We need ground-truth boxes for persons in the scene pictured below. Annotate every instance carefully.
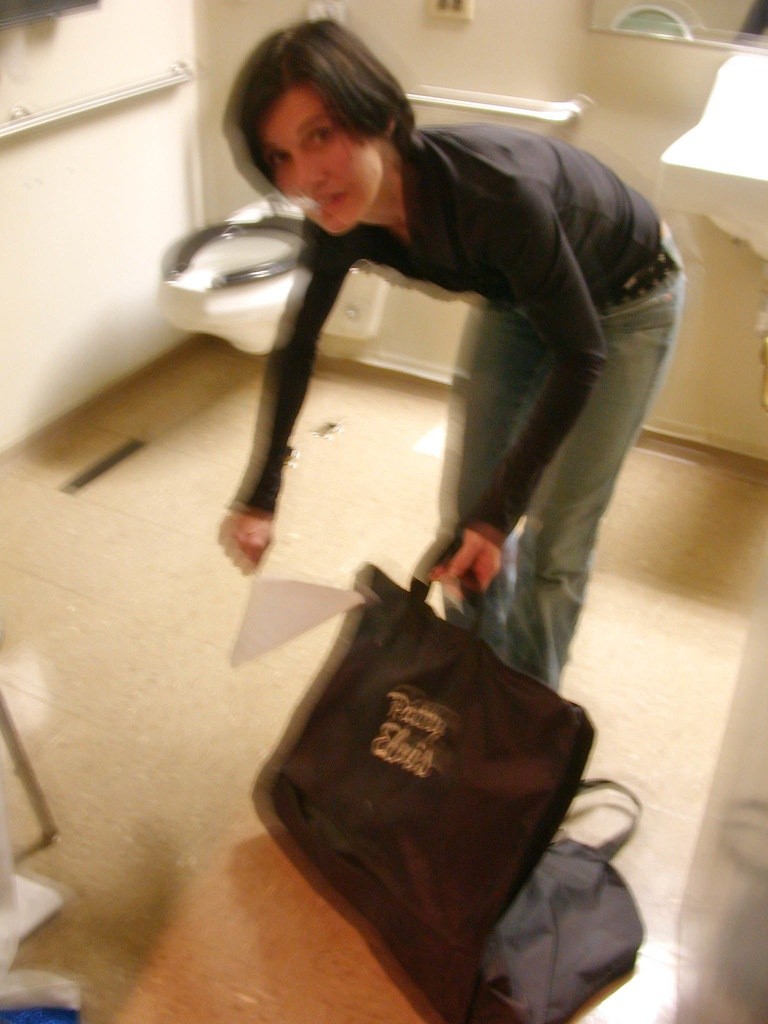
[216,18,685,693]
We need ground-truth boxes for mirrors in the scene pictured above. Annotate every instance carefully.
[590,1,768,55]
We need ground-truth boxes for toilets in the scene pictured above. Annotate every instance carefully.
[154,187,315,357]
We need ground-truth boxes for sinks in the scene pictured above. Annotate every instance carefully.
[653,52,768,265]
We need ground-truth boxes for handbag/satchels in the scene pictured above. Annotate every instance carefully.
[269,541,645,1023]
